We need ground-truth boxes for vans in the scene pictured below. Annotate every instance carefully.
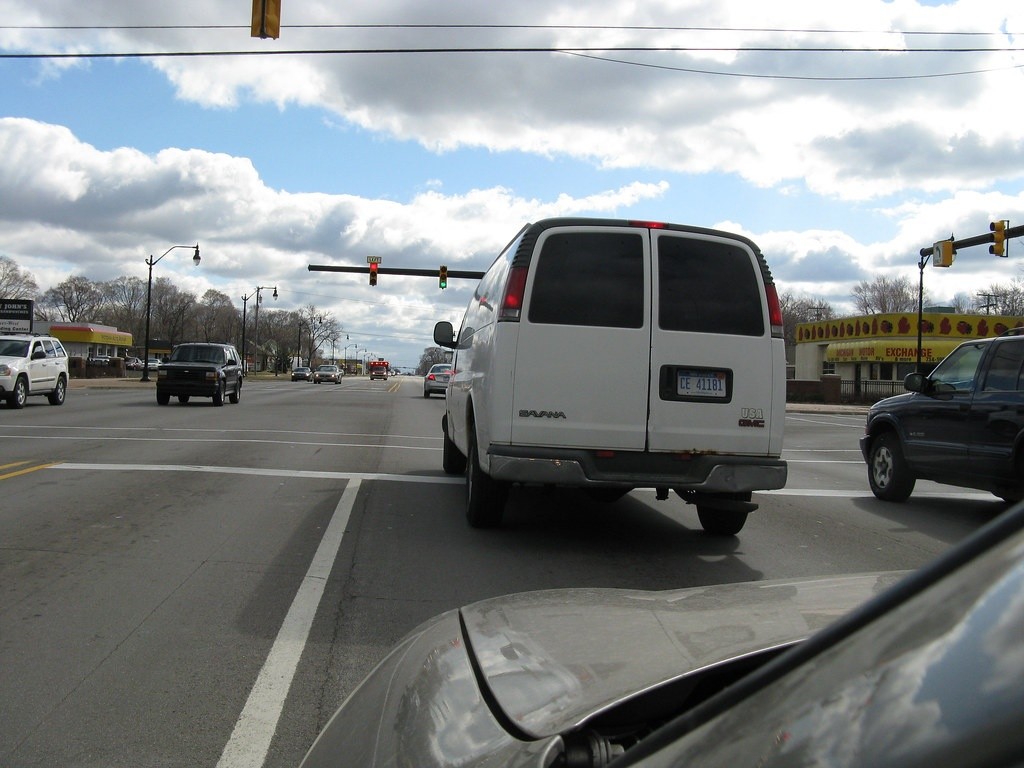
[434,218,788,535]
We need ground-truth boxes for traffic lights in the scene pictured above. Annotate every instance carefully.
[989,221,1005,255]
[370,262,377,285]
[439,266,447,288]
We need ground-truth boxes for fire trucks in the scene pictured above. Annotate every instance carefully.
[369,362,389,380]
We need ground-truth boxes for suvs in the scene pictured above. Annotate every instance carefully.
[0,336,69,408]
[156,343,242,406]
[859,327,1024,502]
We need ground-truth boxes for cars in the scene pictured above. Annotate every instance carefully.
[297,502,1024,768]
[314,365,342,384]
[424,364,452,398]
[93,356,161,371]
[292,367,313,382]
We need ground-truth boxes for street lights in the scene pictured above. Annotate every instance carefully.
[332,334,349,364]
[241,286,278,378]
[140,243,201,381]
[356,349,376,374]
[345,344,357,374]
[297,317,323,367]
